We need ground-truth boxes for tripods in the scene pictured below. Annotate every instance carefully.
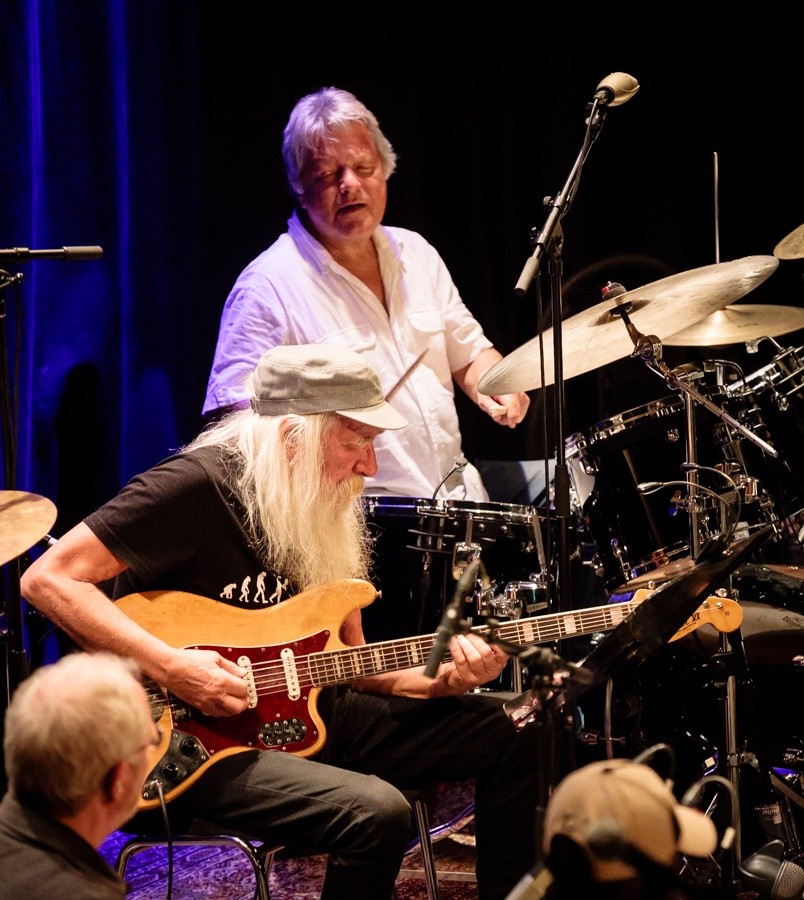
[680,573,804,878]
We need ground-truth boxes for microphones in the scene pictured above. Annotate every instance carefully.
[739,853,804,900]
[423,560,477,680]
[594,72,640,108]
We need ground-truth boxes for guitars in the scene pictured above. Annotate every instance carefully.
[112,578,745,810]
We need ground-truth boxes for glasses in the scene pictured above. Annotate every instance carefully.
[122,727,166,755]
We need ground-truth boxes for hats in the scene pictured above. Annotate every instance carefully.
[543,760,717,881]
[250,343,410,430]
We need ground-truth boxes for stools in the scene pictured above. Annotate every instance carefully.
[115,837,295,900]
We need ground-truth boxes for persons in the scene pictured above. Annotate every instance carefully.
[19,356,557,900]
[0,651,162,900]
[504,758,717,900]
[203,88,529,645]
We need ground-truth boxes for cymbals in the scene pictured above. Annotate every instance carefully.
[773,223,804,260]
[660,305,804,347]
[477,255,780,396]
[0,490,57,567]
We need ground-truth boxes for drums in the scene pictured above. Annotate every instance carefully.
[361,496,558,695]
[555,345,804,808]
